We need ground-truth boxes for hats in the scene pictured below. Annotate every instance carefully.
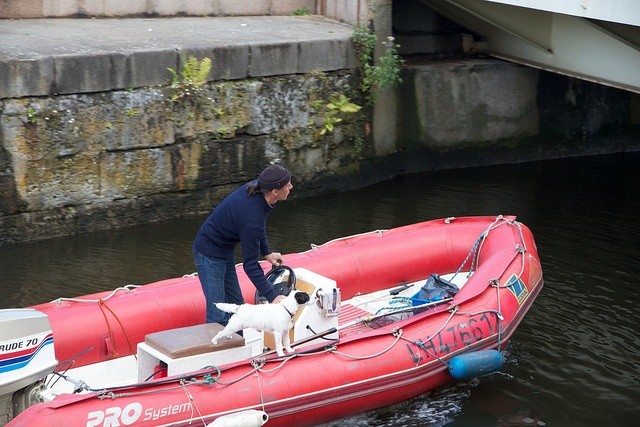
[258,164,290,190]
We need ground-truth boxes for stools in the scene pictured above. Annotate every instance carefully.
[135,322,253,384]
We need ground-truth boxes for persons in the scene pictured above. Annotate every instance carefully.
[192,166,294,324]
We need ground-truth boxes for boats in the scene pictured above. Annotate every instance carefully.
[0,214,544,427]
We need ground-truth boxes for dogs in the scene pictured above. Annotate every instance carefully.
[210,289,318,358]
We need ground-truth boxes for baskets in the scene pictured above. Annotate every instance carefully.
[409,289,447,315]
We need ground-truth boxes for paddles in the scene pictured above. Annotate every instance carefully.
[87,298,456,391]
